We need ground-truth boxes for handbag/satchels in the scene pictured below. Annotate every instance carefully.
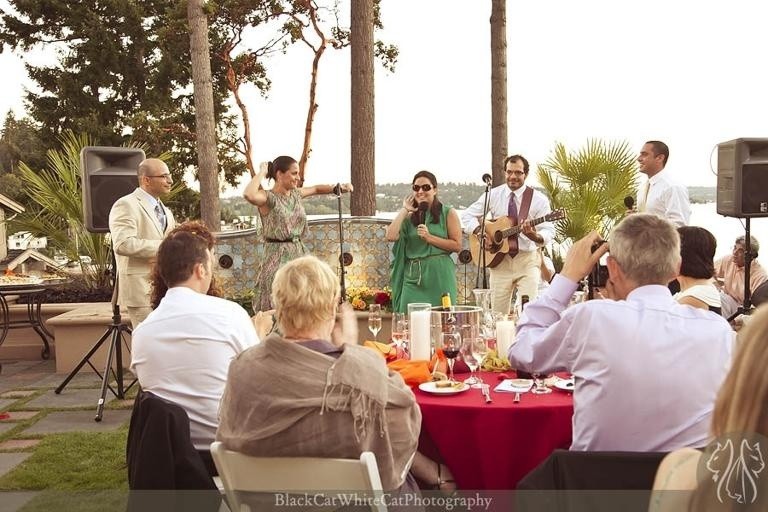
[364,339,396,360]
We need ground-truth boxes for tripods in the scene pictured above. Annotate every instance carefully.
[54,251,138,422]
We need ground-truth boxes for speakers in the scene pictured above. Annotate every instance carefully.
[80,146,147,233]
[717,137,768,217]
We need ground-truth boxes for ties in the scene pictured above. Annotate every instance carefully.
[155,203,164,231]
[640,180,650,212]
[508,191,519,259]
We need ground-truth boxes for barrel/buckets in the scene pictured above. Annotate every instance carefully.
[430,305,484,359]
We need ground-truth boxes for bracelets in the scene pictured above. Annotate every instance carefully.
[402,207,410,213]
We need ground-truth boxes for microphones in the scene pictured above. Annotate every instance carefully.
[419,201,428,240]
[483,174,493,187]
[333,183,343,195]
[624,196,634,210]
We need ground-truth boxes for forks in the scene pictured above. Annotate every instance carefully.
[479,383,494,403]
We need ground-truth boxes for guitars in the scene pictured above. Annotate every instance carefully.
[469,207,566,267]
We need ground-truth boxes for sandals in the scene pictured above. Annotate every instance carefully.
[427,463,462,505]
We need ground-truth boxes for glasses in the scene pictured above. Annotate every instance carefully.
[150,173,173,180]
[412,184,433,192]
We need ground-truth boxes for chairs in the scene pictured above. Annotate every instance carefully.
[126,385,230,511]
[211,441,388,511]
[514,450,711,512]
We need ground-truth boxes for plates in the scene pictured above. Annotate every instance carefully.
[553,379,576,391]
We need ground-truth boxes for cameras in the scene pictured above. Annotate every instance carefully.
[591,241,610,287]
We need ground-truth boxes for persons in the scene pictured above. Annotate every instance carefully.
[147,219,225,308]
[108,158,174,329]
[385,171,462,314]
[129,230,258,474]
[243,155,353,317]
[218,255,457,512]
[462,140,768,512]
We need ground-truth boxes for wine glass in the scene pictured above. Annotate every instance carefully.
[367,287,554,395]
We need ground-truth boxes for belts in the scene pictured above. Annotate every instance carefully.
[267,237,300,244]
